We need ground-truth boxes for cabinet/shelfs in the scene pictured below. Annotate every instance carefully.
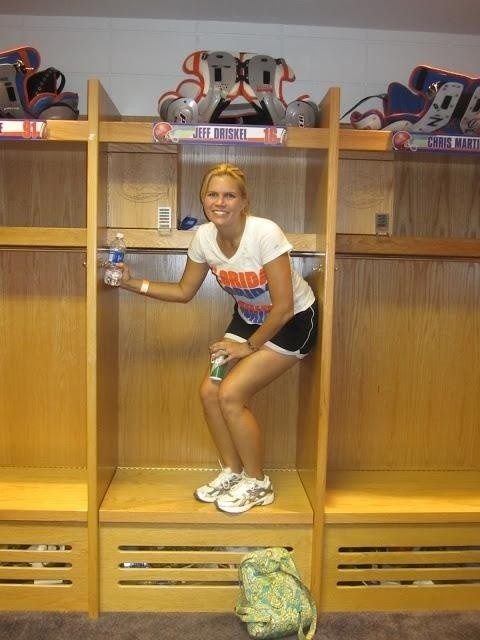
[0,78,480,619]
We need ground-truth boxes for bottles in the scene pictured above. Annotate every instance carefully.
[104,234,126,288]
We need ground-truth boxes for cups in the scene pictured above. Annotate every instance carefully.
[209,347,227,381]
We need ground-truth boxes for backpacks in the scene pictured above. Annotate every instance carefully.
[233,546,318,639]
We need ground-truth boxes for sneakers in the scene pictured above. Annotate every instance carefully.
[193,462,245,504]
[214,473,274,514]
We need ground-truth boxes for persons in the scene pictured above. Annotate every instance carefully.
[101,161,319,517]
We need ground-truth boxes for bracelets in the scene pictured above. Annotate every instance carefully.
[247,337,259,354]
[140,275,150,298]
